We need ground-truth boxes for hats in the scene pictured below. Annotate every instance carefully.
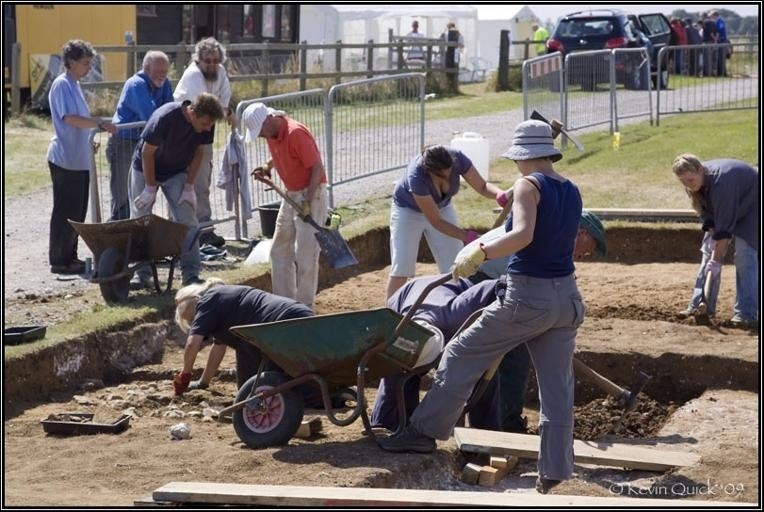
[500,118,563,164]
[241,102,271,144]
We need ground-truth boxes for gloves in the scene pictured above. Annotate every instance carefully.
[173,371,193,396]
[250,163,271,183]
[134,184,157,213]
[187,380,209,392]
[297,199,311,223]
[463,230,481,246]
[450,243,490,283]
[177,183,197,211]
[707,234,729,257]
[495,187,514,208]
[704,259,722,280]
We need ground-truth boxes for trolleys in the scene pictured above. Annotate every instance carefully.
[66,213,217,307]
[218,269,454,452]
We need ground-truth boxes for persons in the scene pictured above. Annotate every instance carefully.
[449,210,607,433]
[385,143,507,298]
[669,11,729,76]
[531,24,549,57]
[104,49,175,221]
[240,101,327,309]
[126,91,225,288]
[672,152,758,327]
[404,21,424,68]
[376,118,585,495]
[173,37,231,249]
[174,278,316,395]
[442,22,464,68]
[361,273,506,435]
[45,39,119,274]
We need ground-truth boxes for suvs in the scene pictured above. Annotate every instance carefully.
[545,7,675,92]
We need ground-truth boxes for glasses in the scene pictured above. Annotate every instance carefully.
[202,58,221,64]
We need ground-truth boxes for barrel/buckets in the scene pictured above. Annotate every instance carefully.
[450,130,490,183]
[257,203,281,238]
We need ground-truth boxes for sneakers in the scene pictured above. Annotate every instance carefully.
[376,422,435,454]
[730,313,757,324]
[535,477,560,494]
[127,270,151,289]
[181,275,205,286]
[679,304,696,318]
[199,231,226,248]
[50,257,87,275]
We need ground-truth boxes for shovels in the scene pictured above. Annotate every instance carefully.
[251,166,360,272]
